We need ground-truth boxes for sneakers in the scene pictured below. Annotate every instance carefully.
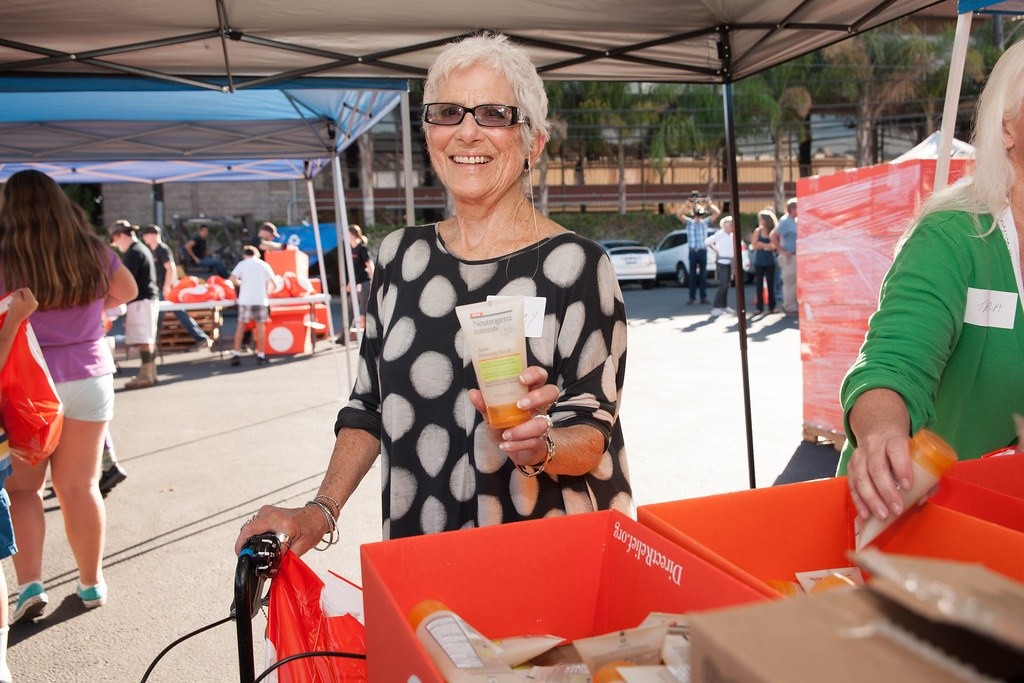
[9,583,49,625]
[100,465,127,499]
[76,581,107,608]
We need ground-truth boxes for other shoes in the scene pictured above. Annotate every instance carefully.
[755,310,774,314]
[257,357,267,366]
[687,299,694,304]
[335,340,345,346]
[126,371,158,388]
[231,356,239,365]
[712,308,736,317]
[786,313,798,317]
[701,300,710,304]
[189,338,213,352]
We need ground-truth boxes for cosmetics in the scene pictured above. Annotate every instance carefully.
[455,296,533,428]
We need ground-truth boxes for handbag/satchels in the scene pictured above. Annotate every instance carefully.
[264,549,367,683]
[0,293,62,464]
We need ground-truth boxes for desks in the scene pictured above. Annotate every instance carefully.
[160,293,329,355]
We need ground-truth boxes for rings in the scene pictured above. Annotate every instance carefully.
[274,533,293,547]
[536,406,547,412]
[534,415,553,434]
[239,516,255,530]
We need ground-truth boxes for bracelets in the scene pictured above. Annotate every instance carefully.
[303,503,333,551]
[314,495,341,522]
[308,501,340,544]
[314,502,334,517]
[515,436,556,477]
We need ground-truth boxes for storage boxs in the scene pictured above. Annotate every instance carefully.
[361,452,1024,683]
[249,278,328,355]
[266,250,310,279]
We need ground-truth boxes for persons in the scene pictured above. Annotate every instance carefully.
[236,31,638,557]
[676,197,798,317]
[838,44,1023,521]
[0,170,376,683]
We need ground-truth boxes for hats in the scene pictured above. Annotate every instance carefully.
[141,224,161,233]
[107,219,140,235]
[260,221,280,239]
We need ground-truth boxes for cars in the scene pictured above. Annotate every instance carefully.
[596,238,657,289]
[648,228,751,286]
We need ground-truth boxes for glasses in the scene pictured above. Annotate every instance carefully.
[110,237,115,243]
[423,102,529,127]
